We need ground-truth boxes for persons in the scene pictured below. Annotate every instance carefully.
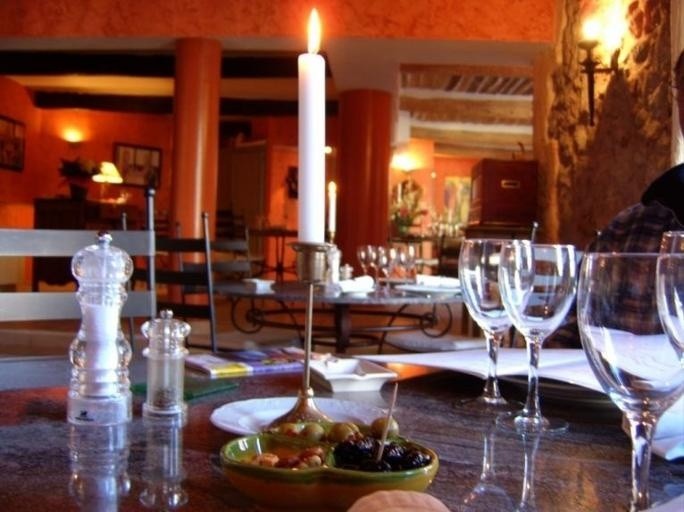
[575,49,684,335]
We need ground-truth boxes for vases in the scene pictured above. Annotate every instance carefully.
[399,224,407,239]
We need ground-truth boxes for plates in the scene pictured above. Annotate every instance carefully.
[298,359,399,395]
[209,392,399,442]
[392,284,459,300]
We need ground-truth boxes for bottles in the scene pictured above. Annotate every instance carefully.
[63,234,134,428]
[66,424,133,512]
[340,263,354,280]
[139,309,193,419]
[137,414,191,511]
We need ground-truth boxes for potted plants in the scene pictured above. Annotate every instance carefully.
[58,155,97,197]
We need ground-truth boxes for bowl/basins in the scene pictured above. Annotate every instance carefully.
[225,420,440,502]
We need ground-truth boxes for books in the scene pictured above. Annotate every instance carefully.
[183,343,319,380]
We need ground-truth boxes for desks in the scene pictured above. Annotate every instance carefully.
[2,370,684,509]
[191,275,461,353]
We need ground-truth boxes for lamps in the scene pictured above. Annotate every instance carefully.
[578,24,620,131]
[94,162,123,191]
[57,131,86,148]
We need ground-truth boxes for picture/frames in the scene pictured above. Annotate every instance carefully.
[1,113,25,172]
[110,143,160,190]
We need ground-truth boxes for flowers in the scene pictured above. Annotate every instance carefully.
[391,204,426,223]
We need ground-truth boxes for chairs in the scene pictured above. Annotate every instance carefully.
[1,193,297,418]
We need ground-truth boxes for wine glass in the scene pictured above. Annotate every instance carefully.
[653,225,683,349]
[354,242,416,295]
[515,429,554,509]
[494,243,579,437]
[454,405,515,512]
[574,249,683,511]
[454,236,531,417]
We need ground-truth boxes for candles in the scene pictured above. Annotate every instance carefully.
[296,9,328,244]
[328,181,338,232]
